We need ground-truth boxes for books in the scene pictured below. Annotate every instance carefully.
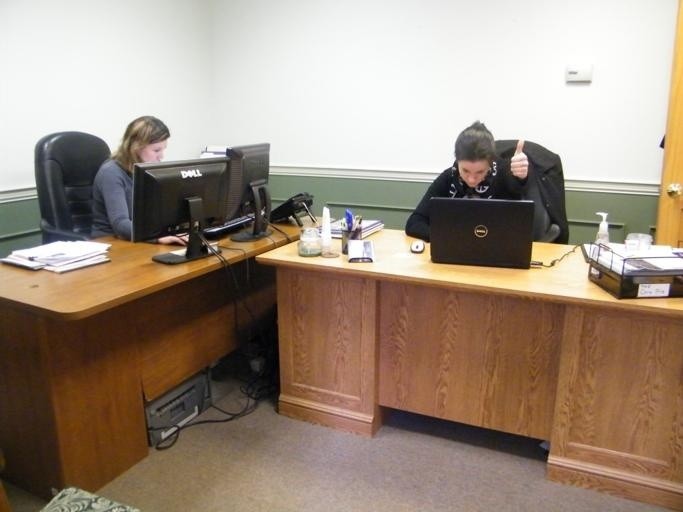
[0,238,114,275]
[315,218,385,240]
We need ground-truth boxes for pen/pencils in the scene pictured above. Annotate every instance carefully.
[342,208,362,252]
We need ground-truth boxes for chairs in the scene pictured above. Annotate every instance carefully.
[33,131,114,247]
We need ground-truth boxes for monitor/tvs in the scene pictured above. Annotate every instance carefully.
[132,155,232,265]
[224,142,273,242]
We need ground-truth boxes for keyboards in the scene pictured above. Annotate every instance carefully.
[203,210,265,239]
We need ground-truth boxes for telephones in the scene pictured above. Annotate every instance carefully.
[270,192,314,221]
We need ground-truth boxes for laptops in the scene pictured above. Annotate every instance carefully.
[428,197,535,269]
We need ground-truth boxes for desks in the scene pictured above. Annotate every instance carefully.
[2,213,336,508]
[254,225,682,511]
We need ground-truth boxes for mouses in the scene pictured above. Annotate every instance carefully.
[411,239,425,252]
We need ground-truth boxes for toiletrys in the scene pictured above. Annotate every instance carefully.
[594,211,610,244]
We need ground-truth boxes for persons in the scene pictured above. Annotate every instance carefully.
[404,120,531,245]
[89,115,191,247]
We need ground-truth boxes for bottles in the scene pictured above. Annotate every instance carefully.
[298,228,321,257]
